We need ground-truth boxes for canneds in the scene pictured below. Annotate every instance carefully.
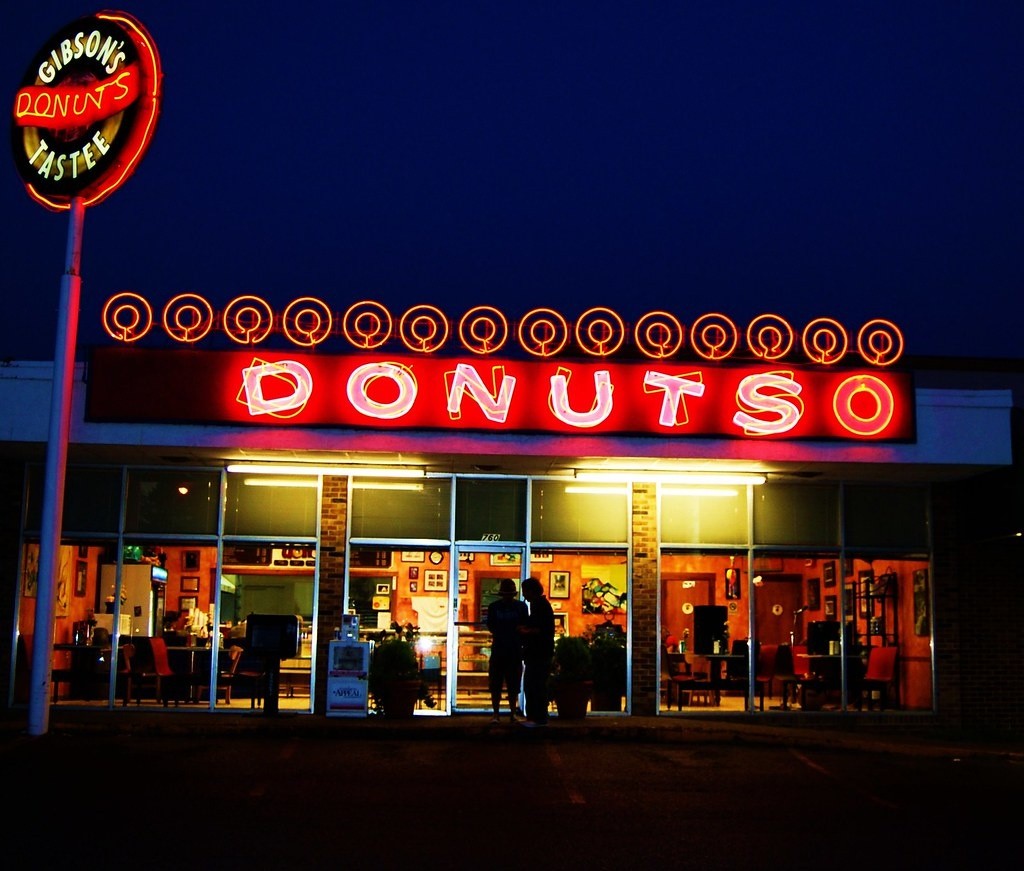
[679,641,685,654]
[829,641,835,656]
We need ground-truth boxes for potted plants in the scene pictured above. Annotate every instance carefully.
[552,630,629,717]
[368,641,439,718]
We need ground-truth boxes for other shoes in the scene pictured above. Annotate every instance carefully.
[490,717,501,727]
[510,717,520,726]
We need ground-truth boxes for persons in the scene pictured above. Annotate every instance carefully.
[516,578,555,728]
[486,578,528,724]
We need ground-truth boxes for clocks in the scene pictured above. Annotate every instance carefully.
[429,552,444,564]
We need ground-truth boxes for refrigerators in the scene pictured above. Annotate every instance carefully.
[98,565,168,640]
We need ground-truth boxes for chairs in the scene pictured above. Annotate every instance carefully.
[663,642,903,713]
[52,637,244,704]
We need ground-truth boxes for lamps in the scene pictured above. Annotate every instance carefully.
[225,464,427,477]
[574,470,768,484]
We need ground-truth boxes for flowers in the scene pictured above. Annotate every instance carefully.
[106,587,127,602]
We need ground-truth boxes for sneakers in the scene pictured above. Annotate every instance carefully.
[520,721,548,727]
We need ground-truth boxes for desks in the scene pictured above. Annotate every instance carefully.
[697,654,743,707]
[166,646,236,703]
[54,644,107,701]
[799,654,868,711]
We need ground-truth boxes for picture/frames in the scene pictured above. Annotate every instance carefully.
[552,612,570,639]
[490,553,522,566]
[182,549,201,572]
[824,595,837,621]
[824,560,836,587]
[549,571,570,599]
[530,553,554,563]
[458,569,468,582]
[858,569,875,617]
[459,553,475,562]
[424,569,448,592]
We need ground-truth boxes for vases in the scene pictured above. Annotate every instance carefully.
[105,602,115,613]
